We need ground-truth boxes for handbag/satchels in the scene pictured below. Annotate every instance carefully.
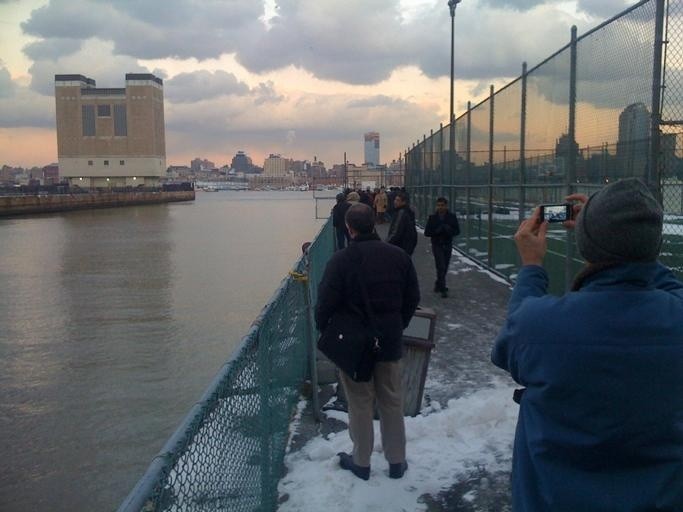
[318,312,379,382]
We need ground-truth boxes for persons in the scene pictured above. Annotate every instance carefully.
[421,195,460,300]
[308,201,427,483]
[483,175,681,509]
[329,179,419,281]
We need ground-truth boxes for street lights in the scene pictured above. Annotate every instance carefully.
[446,0,464,214]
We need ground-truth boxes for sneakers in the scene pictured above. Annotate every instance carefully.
[337,452,369,480]
[434,287,448,298]
[391,461,408,477]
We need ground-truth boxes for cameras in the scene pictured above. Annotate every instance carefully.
[539,202,575,224]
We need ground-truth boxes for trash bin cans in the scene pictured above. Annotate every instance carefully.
[369,305,437,420]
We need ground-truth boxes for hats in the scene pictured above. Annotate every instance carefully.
[575,177,665,261]
[347,192,360,201]
